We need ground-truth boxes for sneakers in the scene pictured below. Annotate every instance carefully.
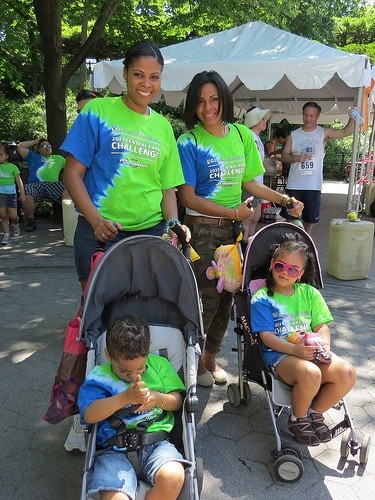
[309,412,332,442]
[11,223,21,237]
[64,413,87,453]
[287,413,320,446]
[2,233,12,245]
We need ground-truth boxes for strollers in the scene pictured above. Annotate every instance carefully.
[76,220,208,499]
[231,198,372,485]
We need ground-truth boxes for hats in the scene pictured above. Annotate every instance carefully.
[244,107,272,128]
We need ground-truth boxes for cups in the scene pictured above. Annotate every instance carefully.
[306,146,312,160]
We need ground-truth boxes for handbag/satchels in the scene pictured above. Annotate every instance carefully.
[263,154,277,175]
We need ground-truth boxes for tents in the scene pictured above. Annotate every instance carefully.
[93,20,375,214]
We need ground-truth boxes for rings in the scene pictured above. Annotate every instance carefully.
[101,233,105,237]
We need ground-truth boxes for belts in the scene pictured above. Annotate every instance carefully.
[183,214,235,227]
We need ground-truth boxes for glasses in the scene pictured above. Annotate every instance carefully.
[76,90,98,97]
[272,257,304,279]
[38,144,52,149]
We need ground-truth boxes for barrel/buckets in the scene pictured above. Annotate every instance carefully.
[327,217,374,280]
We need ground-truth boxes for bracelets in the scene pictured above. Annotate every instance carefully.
[281,193,289,206]
[166,217,180,231]
[234,209,239,220]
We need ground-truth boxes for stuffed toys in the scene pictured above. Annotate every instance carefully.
[287,329,332,365]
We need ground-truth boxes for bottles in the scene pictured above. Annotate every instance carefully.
[347,106,365,125]
[261,208,281,223]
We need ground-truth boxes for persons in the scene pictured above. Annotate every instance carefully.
[75,89,103,115]
[0,138,67,246]
[59,42,191,455]
[178,70,305,387]
[282,102,356,234]
[76,315,187,500]
[248,232,357,447]
[241,102,287,241]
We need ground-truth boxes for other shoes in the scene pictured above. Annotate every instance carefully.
[201,356,228,383]
[243,230,250,243]
[25,219,36,231]
[197,371,215,386]
